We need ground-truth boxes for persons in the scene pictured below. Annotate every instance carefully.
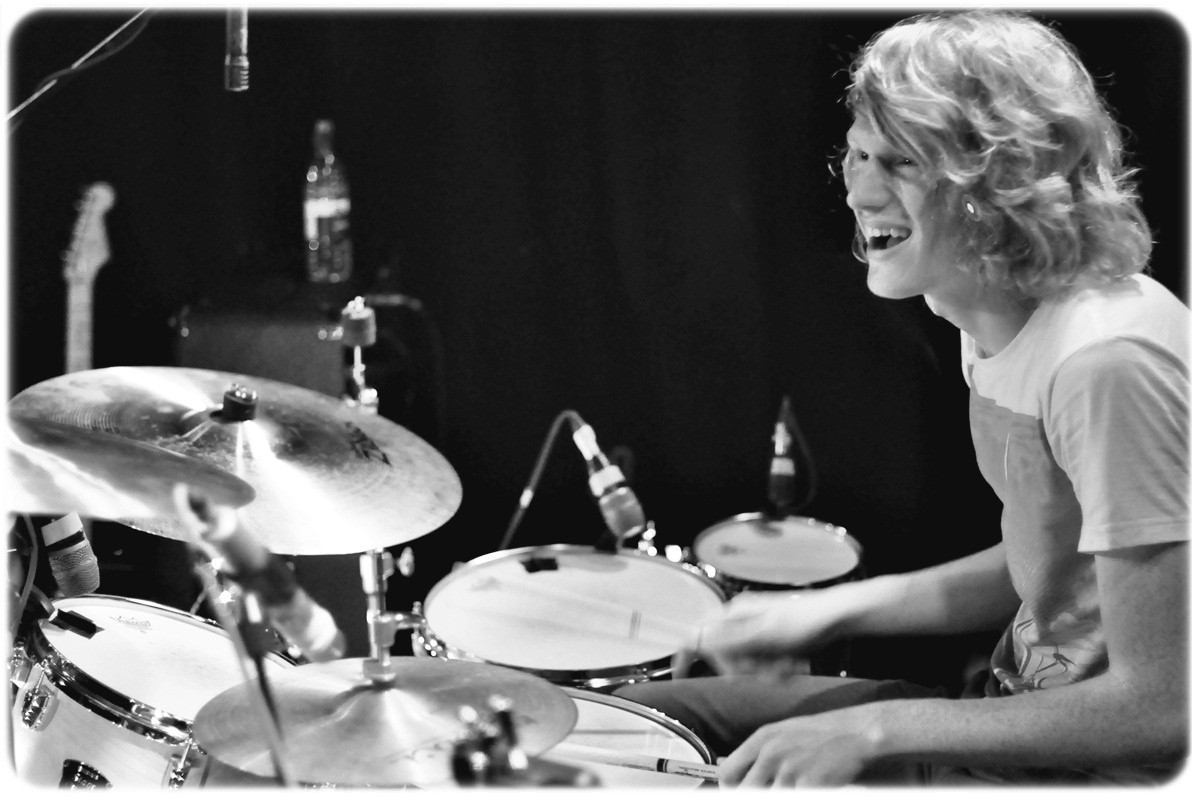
[612,13,1190,786]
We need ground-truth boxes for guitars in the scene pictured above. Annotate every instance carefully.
[61,181,118,372]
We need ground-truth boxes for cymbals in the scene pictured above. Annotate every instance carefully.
[194,647,579,787]
[17,365,466,556]
[3,408,259,523]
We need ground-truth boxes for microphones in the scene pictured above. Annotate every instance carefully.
[186,487,347,660]
[566,412,643,537]
[39,515,100,598]
[226,8,250,92]
[768,404,795,505]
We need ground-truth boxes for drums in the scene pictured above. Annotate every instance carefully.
[485,682,713,791]
[693,510,863,591]
[9,591,301,792]
[414,538,729,687]
